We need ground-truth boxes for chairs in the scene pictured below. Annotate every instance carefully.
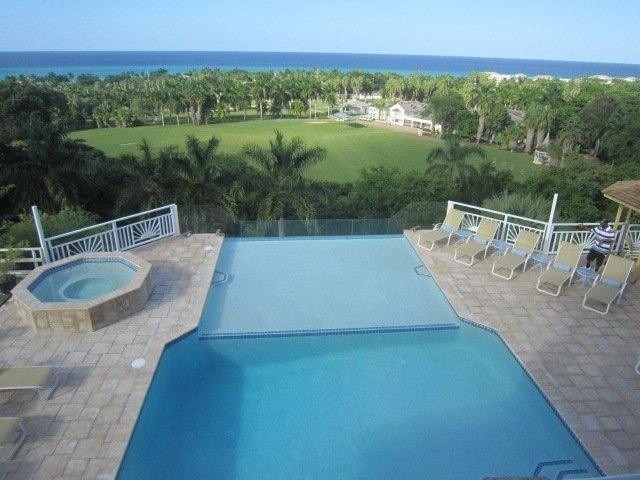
[416,208,635,316]
[0,363,59,463]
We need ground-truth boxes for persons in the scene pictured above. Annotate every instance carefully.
[626,258,640,285]
[577,218,615,271]
